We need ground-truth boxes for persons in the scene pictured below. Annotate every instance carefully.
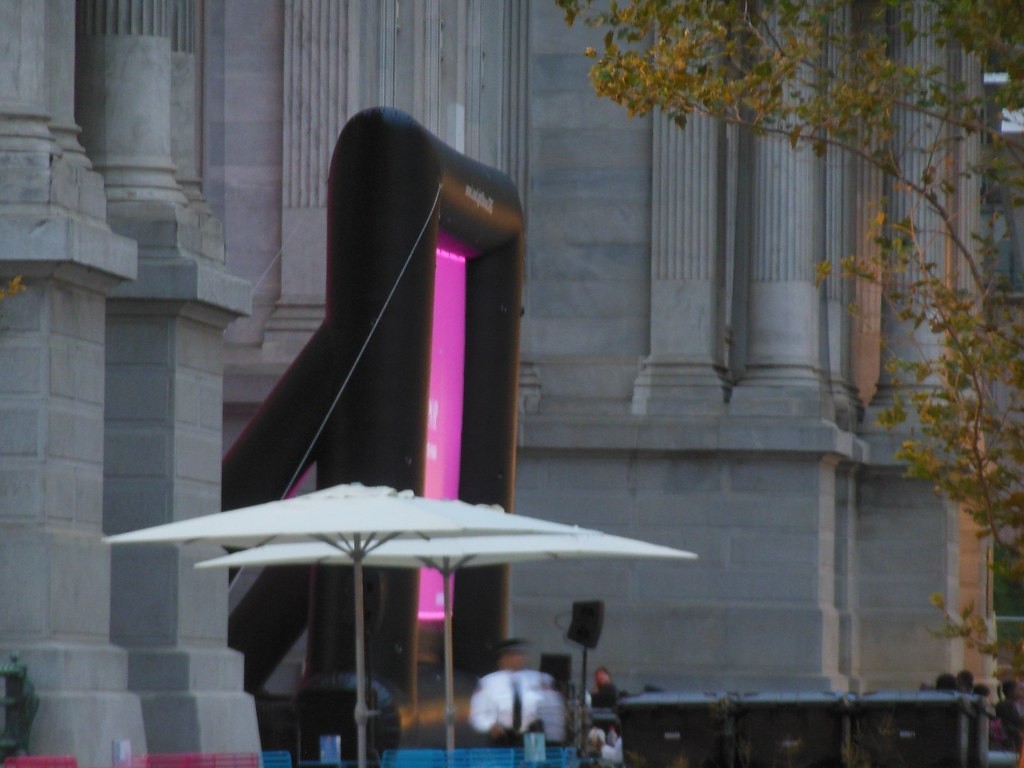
[584,669,623,767]
[468,638,558,748]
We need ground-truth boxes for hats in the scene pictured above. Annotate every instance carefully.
[495,638,530,654]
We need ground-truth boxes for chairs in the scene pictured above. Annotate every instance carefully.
[4,746,595,768]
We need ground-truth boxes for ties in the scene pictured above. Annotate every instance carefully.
[513,680,521,731]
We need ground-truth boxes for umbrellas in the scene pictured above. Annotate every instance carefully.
[192,530,700,767]
[103,481,587,767]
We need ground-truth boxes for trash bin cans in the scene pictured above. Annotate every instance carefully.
[621,691,990,768]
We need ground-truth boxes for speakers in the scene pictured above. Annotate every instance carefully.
[567,599,604,650]
[539,652,570,683]
